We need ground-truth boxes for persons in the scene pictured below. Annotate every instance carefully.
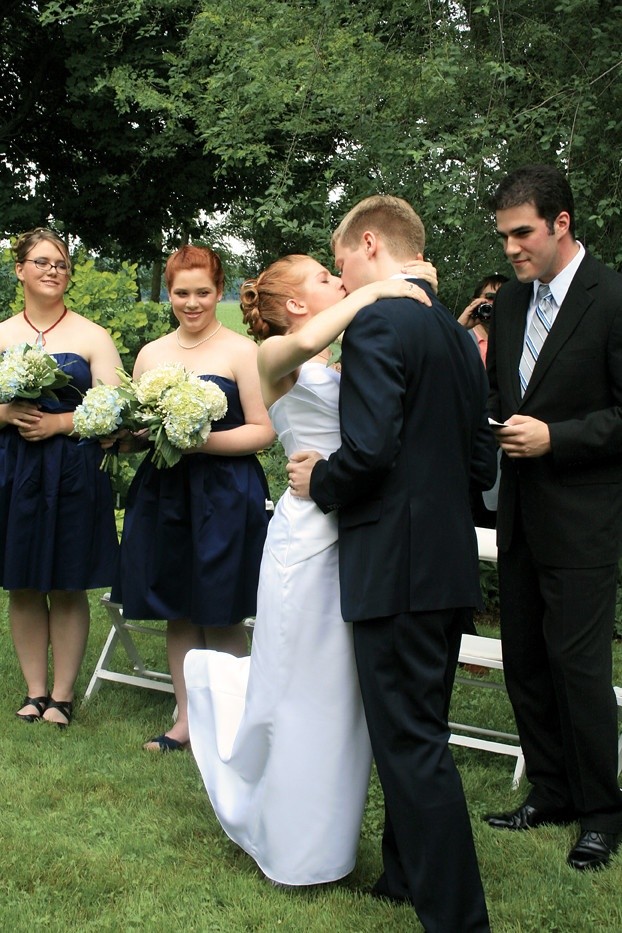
[284,194,498,933]
[456,274,504,529]
[457,619,490,678]
[482,165,622,867]
[0,228,126,727]
[183,254,439,886]
[100,245,275,754]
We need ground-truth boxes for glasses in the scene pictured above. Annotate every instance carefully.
[23,257,71,275]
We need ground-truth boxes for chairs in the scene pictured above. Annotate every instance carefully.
[79,500,526,790]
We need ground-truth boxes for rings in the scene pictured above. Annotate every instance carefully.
[408,285,413,290]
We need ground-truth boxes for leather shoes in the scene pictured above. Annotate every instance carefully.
[567,828,618,869]
[482,802,573,830]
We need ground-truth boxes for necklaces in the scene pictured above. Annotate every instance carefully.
[23,306,68,348]
[176,324,223,350]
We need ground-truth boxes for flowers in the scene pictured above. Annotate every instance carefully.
[0,331,227,475]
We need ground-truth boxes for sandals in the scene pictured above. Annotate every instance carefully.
[143,732,189,753]
[15,688,74,729]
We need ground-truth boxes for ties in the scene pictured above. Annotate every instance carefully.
[519,284,553,401]
